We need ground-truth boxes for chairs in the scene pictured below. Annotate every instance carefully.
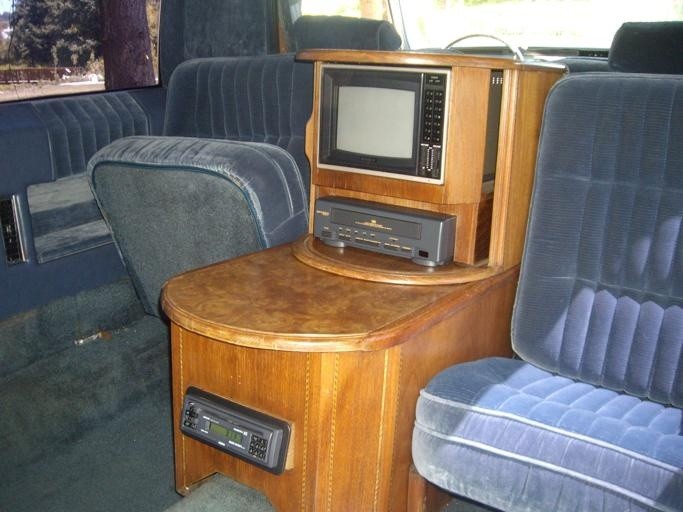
[87,134,307,316]
[159,51,316,190]
[287,14,401,58]
[407,21,683,511]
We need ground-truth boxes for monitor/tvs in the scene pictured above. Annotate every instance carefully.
[317,64,450,186]
